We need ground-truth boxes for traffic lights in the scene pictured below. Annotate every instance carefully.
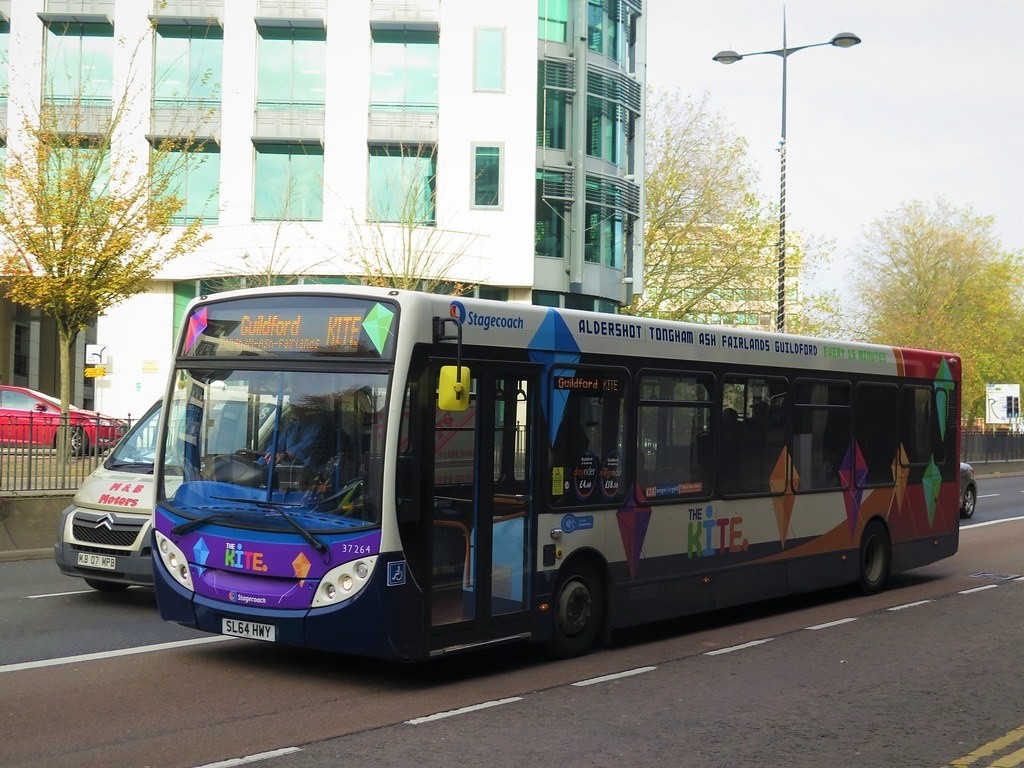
[1006,396,1012,417]
[1013,397,1019,417]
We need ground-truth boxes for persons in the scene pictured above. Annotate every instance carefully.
[722,400,790,449]
[255,391,327,469]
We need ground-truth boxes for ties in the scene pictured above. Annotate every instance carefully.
[292,425,304,444]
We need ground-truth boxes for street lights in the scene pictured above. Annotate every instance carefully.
[712,3,862,335]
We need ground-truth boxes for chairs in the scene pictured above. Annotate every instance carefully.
[691,429,741,489]
[310,412,353,471]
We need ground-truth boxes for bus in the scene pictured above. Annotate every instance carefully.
[150,283,962,665]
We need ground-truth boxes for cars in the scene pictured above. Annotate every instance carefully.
[0,385,127,457]
[960,462,978,518]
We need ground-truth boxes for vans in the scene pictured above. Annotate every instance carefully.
[55,385,475,593]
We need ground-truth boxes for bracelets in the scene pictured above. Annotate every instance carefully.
[282,451,288,459]
